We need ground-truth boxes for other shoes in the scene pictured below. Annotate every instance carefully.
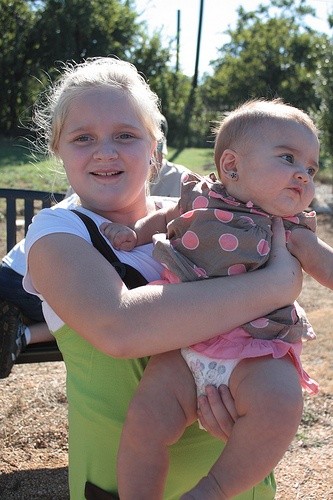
[16,324,26,355]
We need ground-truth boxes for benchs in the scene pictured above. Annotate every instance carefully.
[0,188,66,365]
